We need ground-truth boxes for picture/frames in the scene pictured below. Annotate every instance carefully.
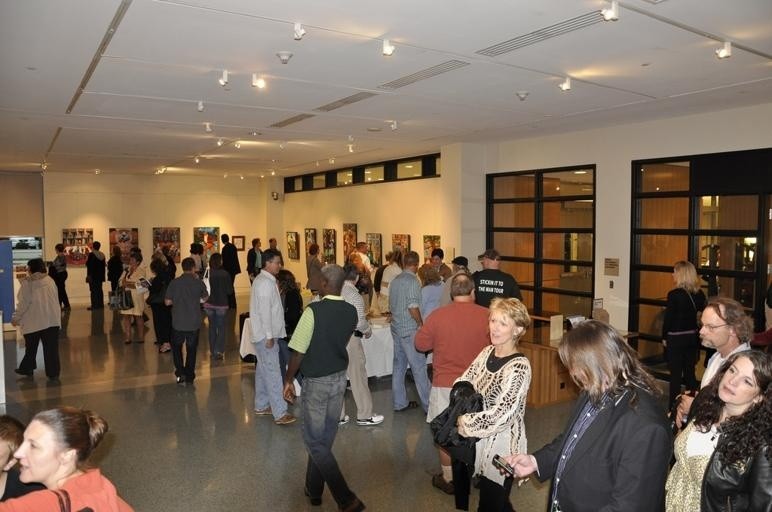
[232,234,246,253]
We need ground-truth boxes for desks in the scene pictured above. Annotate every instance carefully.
[360,317,435,379]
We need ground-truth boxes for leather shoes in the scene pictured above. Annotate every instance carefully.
[47,375,58,381]
[14,368,34,376]
[120,314,225,362]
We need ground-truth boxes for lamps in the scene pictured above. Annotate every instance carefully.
[276,52,292,65]
[219,71,229,87]
[294,23,306,40]
[517,92,529,102]
[252,74,266,89]
[382,40,395,56]
[223,170,276,181]
[391,121,400,134]
[198,102,206,113]
[601,2,620,22]
[316,145,353,167]
[558,78,572,92]
[206,123,241,149]
[157,166,168,177]
[194,157,200,165]
[716,42,732,59]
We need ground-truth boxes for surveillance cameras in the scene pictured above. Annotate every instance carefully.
[271,192,278,200]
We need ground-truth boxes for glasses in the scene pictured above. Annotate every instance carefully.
[700,322,733,331]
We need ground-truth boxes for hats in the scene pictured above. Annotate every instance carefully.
[478,249,501,262]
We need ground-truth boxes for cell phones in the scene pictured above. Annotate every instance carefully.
[494,454,514,475]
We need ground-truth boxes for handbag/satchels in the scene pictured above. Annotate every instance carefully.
[200,267,211,303]
[108,279,135,311]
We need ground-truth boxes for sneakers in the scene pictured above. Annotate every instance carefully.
[431,472,455,495]
[338,414,350,425]
[176,373,194,386]
[394,401,420,412]
[338,497,366,512]
[303,485,322,506]
[253,407,273,415]
[356,412,385,426]
[273,414,298,425]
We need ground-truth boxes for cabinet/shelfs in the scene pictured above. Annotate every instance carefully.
[510,325,644,411]
